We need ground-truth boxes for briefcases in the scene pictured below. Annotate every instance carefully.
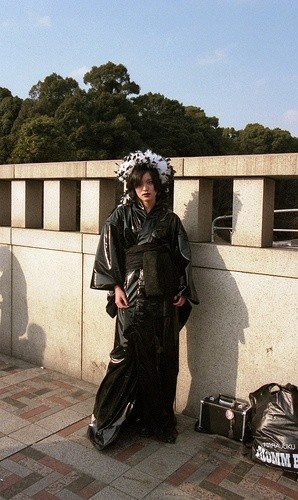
[200,392,251,442]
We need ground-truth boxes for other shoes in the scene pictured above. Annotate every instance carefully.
[139,422,152,437]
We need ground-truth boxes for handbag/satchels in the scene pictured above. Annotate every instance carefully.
[249,384,298,474]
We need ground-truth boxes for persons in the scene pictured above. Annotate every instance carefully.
[87,152,199,452]
